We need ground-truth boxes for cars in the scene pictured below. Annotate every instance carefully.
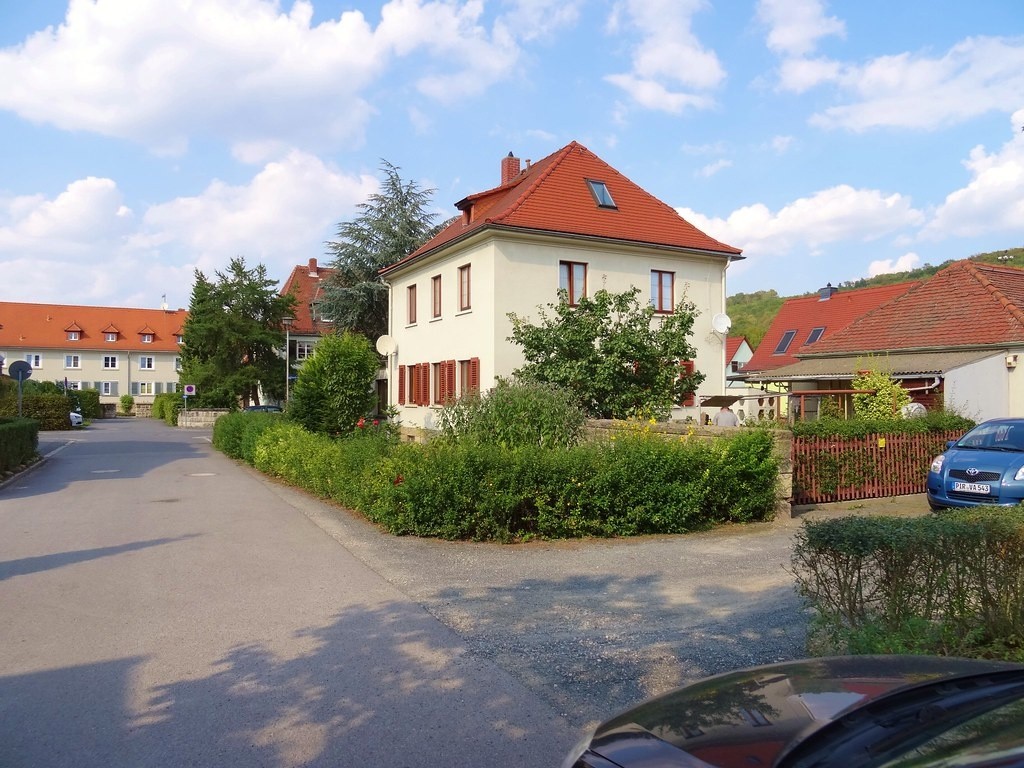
[241,406,282,412]
[927,418,1024,510]
[573,656,1024,768]
[70,413,84,427]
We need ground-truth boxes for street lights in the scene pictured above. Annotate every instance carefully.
[280,316,297,405]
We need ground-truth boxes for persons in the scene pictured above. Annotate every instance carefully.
[712,407,738,427]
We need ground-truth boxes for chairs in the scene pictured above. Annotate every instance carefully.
[1004,428,1024,447]
